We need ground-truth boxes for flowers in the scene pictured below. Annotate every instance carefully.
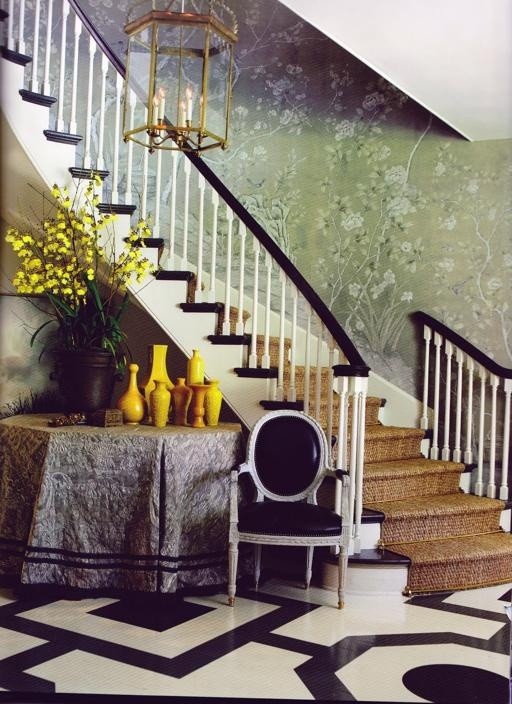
[1,171,166,376]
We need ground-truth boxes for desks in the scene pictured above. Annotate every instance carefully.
[0,409,244,596]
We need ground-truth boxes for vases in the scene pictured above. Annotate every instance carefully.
[75,343,224,428]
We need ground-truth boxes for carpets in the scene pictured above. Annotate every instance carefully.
[129,204,512,598]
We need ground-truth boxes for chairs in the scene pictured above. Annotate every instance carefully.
[225,407,351,610]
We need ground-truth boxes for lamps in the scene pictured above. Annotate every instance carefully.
[120,0,241,160]
